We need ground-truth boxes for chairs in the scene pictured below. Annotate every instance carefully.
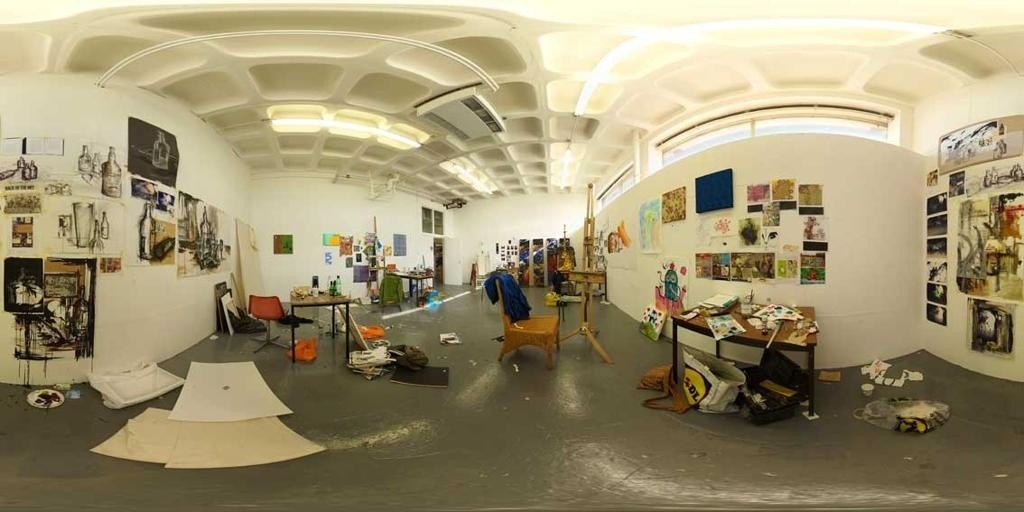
[496,278,560,371]
[248,295,290,354]
[553,270,589,323]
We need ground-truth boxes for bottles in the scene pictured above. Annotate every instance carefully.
[981,121,1023,188]
[329,274,341,297]
[407,265,424,277]
[760,316,769,335]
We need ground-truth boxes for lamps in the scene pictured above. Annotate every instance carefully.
[416,86,506,143]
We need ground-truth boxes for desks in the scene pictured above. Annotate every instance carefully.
[670,302,817,416]
[290,288,354,363]
[567,271,607,302]
[386,272,434,306]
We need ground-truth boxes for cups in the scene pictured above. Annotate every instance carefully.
[312,287,319,298]
[862,384,875,397]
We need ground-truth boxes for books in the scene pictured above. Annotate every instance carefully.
[700,295,738,313]
[390,365,450,387]
[346,349,392,380]
